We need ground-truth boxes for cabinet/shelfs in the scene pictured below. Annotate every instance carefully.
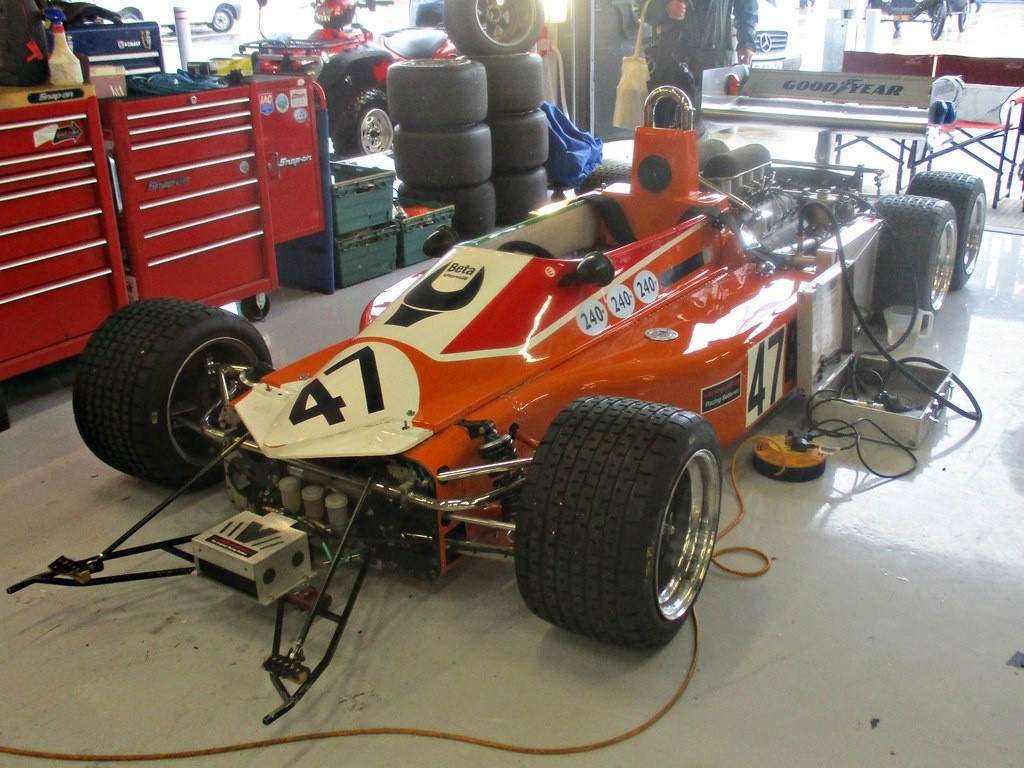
[0,68,326,432]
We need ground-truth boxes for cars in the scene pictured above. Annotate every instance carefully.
[96,0,242,34]
[730,0,802,72]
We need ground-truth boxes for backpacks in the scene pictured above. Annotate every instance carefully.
[538,100,603,187]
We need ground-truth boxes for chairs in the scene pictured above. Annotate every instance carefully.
[907,54,1024,208]
[828,50,933,194]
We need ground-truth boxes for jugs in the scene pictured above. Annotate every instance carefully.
[882,306,934,348]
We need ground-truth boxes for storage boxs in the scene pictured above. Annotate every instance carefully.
[393,198,456,267]
[330,161,398,235]
[89,66,127,98]
[330,222,401,289]
[795,259,955,450]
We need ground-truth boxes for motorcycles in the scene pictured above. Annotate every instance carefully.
[231,0,463,160]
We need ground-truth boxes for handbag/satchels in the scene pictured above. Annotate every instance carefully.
[612,56,650,129]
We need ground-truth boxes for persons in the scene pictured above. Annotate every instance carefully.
[632,0,759,140]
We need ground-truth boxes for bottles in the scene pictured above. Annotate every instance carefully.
[48,25,84,86]
